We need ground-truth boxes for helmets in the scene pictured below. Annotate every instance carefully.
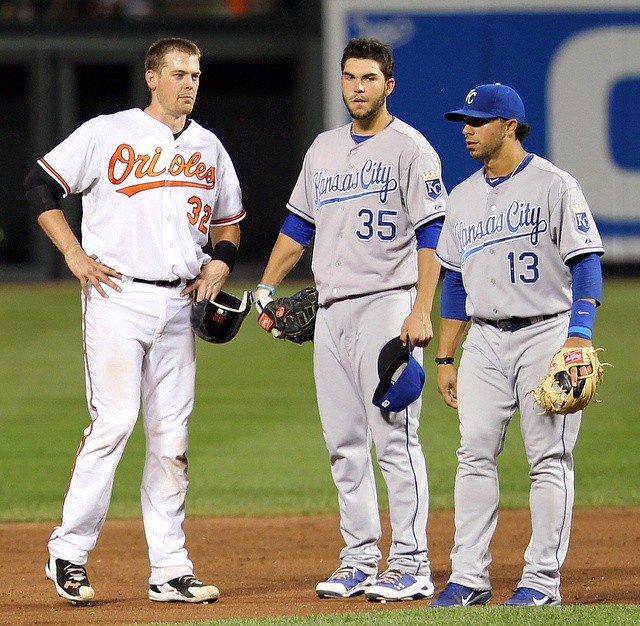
[190,291,250,343]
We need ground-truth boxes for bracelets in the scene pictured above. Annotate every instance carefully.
[257,284,275,295]
[437,362,453,366]
[568,299,596,340]
[211,240,237,274]
[435,357,454,362]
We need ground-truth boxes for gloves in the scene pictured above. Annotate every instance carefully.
[250,286,285,338]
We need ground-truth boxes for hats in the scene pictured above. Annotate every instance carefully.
[371,334,425,413]
[443,83,526,126]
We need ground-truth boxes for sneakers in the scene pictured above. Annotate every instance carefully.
[504,587,561,607]
[316,567,379,598]
[149,575,221,603]
[428,584,492,607]
[45,554,94,607]
[365,569,434,603]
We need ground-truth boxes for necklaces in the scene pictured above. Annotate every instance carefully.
[485,152,528,195]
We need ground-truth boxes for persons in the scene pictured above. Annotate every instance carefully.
[249,37,449,603]
[23,37,250,604]
[428,82,605,609]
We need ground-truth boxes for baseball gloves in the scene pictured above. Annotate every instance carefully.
[249,287,318,344]
[524,346,615,415]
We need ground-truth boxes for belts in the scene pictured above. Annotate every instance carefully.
[105,267,201,288]
[473,308,573,332]
